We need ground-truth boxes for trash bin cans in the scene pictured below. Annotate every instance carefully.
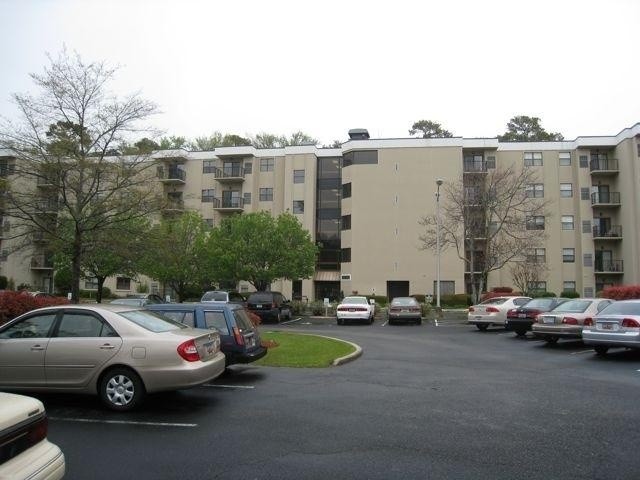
[425,294,433,304]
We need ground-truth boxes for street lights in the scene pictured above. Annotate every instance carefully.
[436,179,444,324]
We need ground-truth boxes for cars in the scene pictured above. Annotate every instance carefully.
[388,297,423,325]
[582,298,640,356]
[506,296,572,338]
[531,297,618,344]
[110,290,293,365]
[0,301,227,413]
[336,295,375,325]
[0,391,67,479]
[467,296,533,331]
